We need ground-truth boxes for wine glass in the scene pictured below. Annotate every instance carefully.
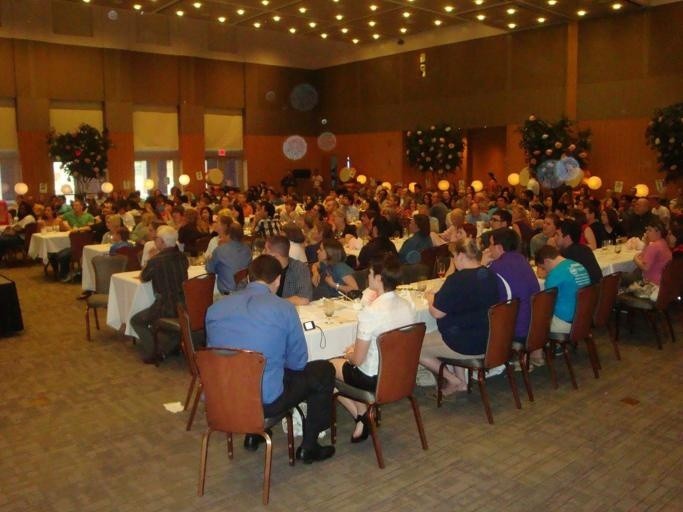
[415,276,427,303]
[436,261,445,283]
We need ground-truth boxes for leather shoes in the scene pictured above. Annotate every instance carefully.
[245,428,272,451]
[296,446,333,463]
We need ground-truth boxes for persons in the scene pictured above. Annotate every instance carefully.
[0,172,683,464]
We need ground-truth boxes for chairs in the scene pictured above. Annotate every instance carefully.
[21,222,683,504]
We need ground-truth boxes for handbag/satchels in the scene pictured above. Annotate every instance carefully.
[631,281,655,298]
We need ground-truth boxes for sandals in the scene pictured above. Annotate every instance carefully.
[352,416,368,442]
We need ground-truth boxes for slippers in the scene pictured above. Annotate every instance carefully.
[425,389,452,402]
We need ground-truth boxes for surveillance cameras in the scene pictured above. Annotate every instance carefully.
[397,38,404,45]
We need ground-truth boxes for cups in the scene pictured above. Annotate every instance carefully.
[602,238,620,255]
[322,297,334,318]
[183,251,207,266]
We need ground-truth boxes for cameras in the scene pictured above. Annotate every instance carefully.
[303,321,315,330]
